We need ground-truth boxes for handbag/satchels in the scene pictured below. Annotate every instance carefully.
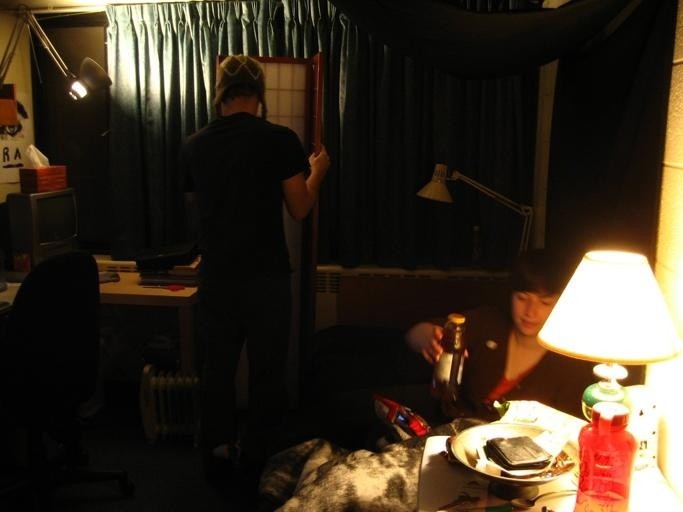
[484,434,555,472]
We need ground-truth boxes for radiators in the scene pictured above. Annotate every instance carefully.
[136,362,214,447]
[314,263,496,334]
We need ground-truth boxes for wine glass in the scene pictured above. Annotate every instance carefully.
[450,422,579,501]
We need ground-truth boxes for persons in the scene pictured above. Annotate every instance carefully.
[178,53,330,465]
[404,249,592,416]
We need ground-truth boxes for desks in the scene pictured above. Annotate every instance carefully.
[0,259,203,376]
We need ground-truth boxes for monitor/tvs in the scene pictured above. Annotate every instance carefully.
[0,190,78,270]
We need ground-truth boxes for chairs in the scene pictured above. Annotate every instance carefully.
[1,244,139,512]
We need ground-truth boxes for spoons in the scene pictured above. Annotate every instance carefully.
[508,490,559,510]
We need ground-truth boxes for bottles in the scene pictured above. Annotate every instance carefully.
[430,312,468,404]
[573,400,637,512]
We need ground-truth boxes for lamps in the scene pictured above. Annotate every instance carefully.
[415,160,535,255]
[536,246,679,481]
[1,2,115,103]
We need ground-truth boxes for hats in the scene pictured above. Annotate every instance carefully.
[211,52,268,108]
